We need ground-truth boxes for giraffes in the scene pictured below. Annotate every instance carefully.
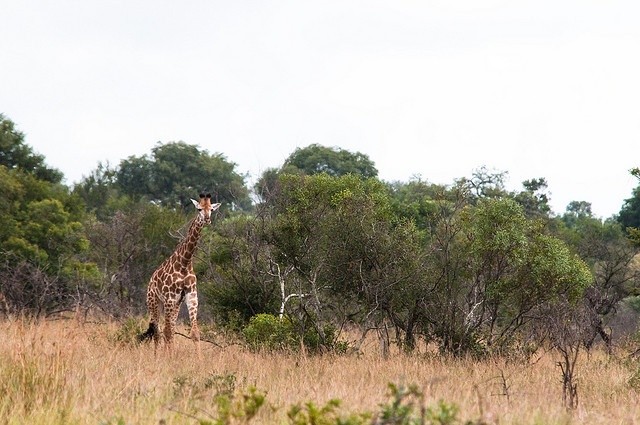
[137,193,223,369]
